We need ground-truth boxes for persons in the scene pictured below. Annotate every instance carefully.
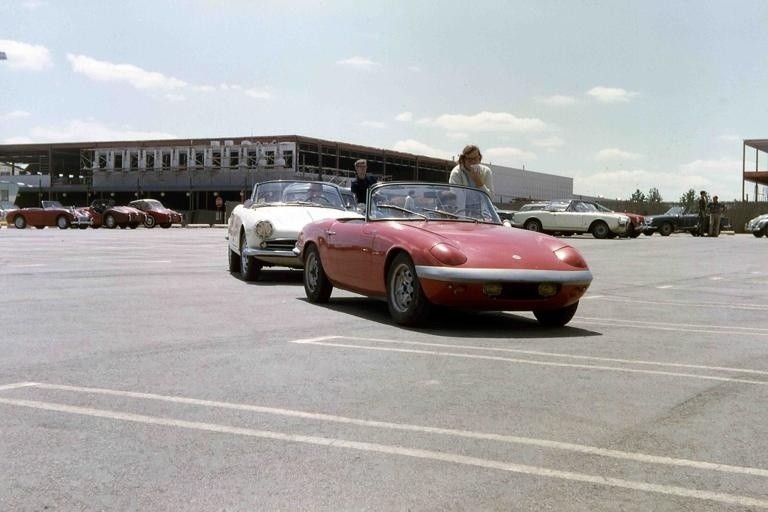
[350,159,376,218]
[449,145,495,220]
[704,196,726,237]
[696,190,708,237]
[436,192,458,219]
[305,183,327,205]
[402,190,416,217]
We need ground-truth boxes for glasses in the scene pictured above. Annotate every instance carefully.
[463,155,479,162]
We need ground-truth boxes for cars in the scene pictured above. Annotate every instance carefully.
[5,199,183,229]
[292,181,593,328]
[224,180,367,282]
[494,199,768,239]
